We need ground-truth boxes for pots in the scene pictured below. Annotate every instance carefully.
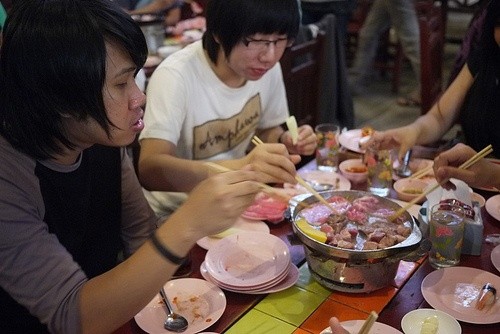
[289,190,423,259]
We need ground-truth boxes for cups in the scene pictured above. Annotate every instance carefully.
[429,202,466,269]
[314,124,340,172]
[365,141,392,197]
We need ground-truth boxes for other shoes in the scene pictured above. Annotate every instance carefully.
[398,91,422,106]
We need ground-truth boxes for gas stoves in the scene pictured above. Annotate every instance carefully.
[302,242,411,293]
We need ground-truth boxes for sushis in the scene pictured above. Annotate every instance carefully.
[477,283,497,311]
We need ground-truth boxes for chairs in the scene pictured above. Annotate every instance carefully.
[283,0,486,131]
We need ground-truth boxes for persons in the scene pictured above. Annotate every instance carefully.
[0,0,500,334]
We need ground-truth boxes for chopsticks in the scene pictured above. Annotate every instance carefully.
[202,160,310,209]
[251,135,340,217]
[390,144,493,221]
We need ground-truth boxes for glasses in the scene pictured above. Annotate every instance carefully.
[243,38,295,50]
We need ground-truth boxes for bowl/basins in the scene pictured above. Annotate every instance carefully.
[393,179,429,204]
[339,159,369,186]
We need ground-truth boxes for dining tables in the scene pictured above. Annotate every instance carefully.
[113,145,500,334]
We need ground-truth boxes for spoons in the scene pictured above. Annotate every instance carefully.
[159,287,188,331]
[395,148,413,178]
[302,177,338,191]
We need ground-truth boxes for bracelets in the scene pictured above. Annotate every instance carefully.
[151,227,187,265]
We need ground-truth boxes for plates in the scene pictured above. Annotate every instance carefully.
[338,128,370,153]
[470,158,500,276]
[392,158,433,181]
[196,190,299,293]
[421,267,500,324]
[133,277,227,334]
[283,170,351,196]
[318,309,461,334]
